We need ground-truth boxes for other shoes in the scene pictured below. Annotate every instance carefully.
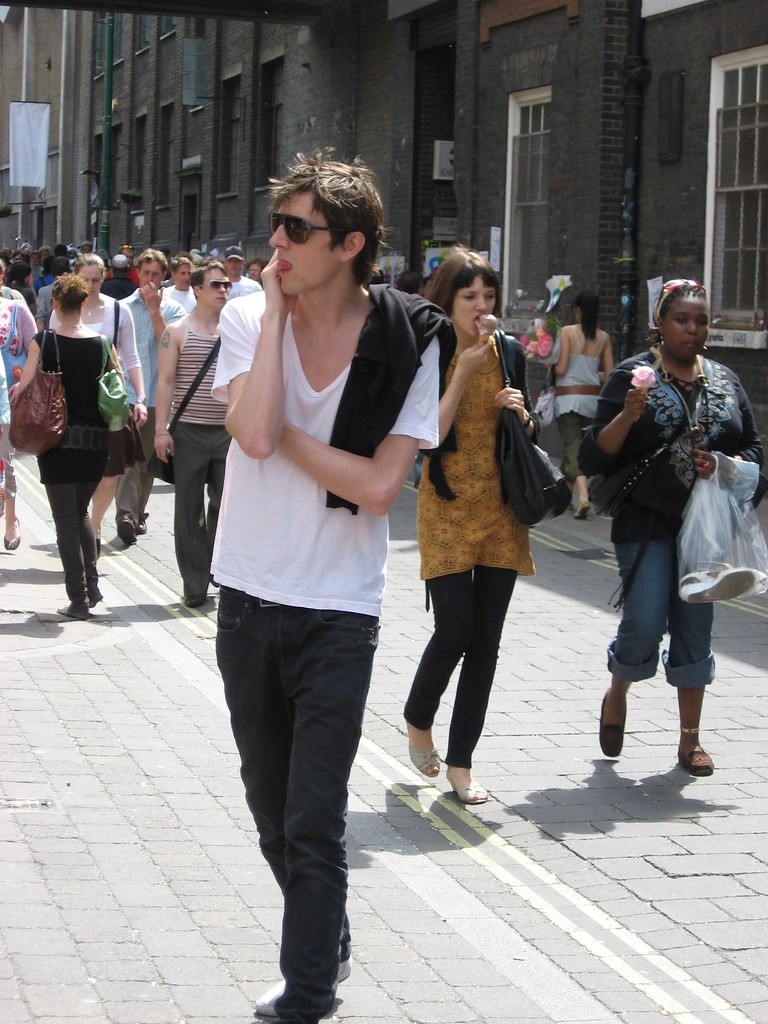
[600,694,626,757]
[185,594,206,607]
[134,519,146,535]
[4,535,20,550]
[678,747,713,776]
[118,522,137,545]
[338,960,351,982]
[254,1000,282,1024]
[57,600,90,621]
[89,595,103,608]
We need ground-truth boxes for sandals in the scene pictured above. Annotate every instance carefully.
[406,720,440,777]
[447,766,488,804]
[679,564,753,603]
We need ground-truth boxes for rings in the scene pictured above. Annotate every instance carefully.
[703,460,713,471]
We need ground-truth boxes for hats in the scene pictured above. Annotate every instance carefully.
[112,254,128,268]
[225,246,244,260]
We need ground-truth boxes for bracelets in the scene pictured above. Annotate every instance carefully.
[524,416,532,427]
[155,433,170,436]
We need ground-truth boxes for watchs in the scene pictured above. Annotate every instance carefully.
[136,397,147,406]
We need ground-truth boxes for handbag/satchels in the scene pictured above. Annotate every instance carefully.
[676,450,768,603]
[579,428,650,519]
[99,338,129,431]
[8,330,66,455]
[495,331,560,527]
[148,452,175,483]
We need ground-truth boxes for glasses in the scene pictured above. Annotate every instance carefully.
[268,211,351,244]
[198,280,232,291]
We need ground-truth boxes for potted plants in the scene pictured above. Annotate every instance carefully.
[120,187,143,204]
[0,201,13,218]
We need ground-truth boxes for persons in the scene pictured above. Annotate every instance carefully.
[155,259,232,606]
[0,234,271,318]
[48,255,149,565]
[395,268,429,490]
[0,261,39,550]
[543,291,615,519]
[17,275,125,618]
[210,148,458,1024]
[577,274,768,776]
[117,249,188,545]
[403,245,543,804]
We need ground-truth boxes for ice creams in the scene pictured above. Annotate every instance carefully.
[630,365,656,395]
[475,314,497,343]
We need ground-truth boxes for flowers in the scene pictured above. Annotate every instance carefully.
[519,314,565,360]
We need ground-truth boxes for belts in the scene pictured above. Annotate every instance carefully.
[556,385,601,395]
